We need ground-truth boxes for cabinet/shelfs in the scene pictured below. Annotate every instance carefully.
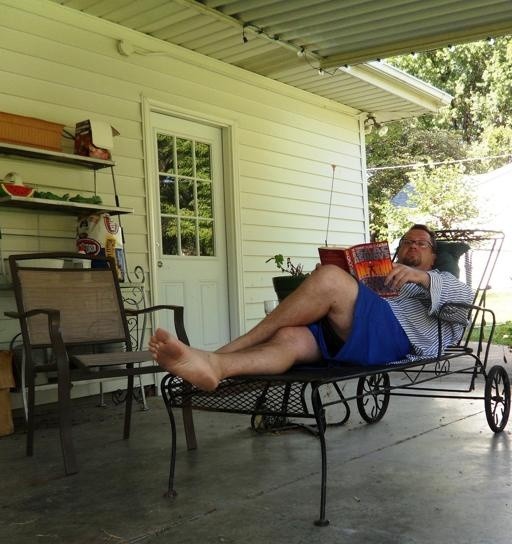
[1,142,148,422]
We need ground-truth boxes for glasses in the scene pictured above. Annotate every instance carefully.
[399,239,434,250]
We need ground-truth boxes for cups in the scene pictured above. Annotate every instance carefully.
[264,300,279,314]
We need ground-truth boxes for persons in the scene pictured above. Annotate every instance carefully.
[149,223,475,394]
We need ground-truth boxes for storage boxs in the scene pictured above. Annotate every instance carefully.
[1,110,67,151]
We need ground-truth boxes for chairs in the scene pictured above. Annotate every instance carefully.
[5,251,200,476]
[162,228,512,528]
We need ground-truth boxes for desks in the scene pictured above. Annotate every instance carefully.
[251,300,351,442]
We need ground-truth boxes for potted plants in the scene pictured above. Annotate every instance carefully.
[265,253,306,304]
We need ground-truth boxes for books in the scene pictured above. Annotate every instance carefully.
[317,240,399,297]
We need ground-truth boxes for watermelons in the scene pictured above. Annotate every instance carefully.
[1,184,36,198]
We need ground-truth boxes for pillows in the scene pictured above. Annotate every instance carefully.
[394,240,472,281]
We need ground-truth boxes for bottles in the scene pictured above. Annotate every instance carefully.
[72,247,92,280]
